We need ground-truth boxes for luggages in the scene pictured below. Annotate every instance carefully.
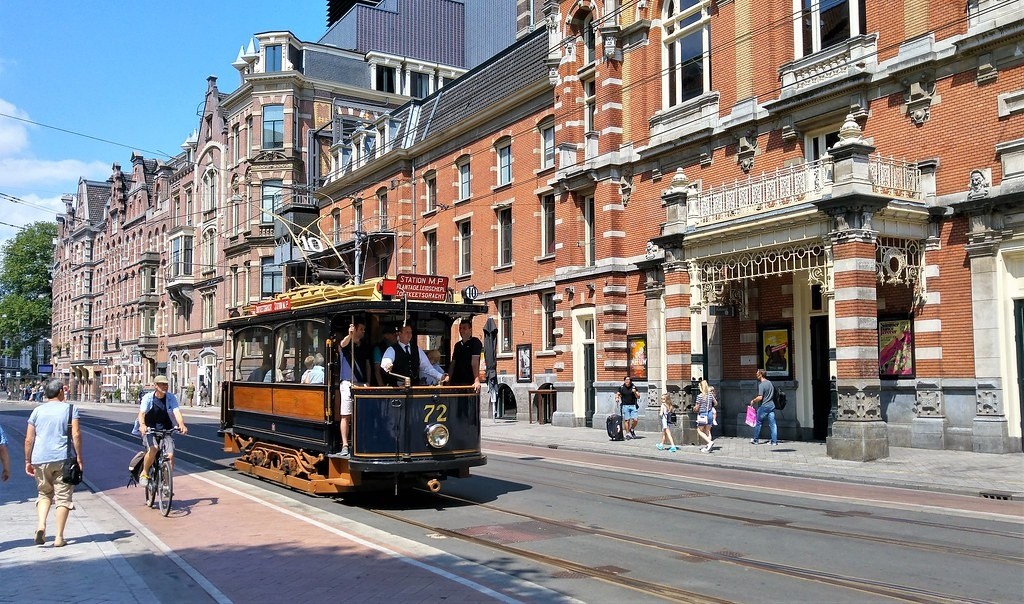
[607,401,624,441]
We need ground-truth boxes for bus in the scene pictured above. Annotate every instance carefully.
[217,182,490,503]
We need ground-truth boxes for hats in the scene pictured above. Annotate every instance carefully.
[154,376,169,384]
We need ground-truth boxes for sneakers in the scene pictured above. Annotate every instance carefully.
[162,486,170,496]
[139,472,149,487]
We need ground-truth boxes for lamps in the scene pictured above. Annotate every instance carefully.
[565,286,574,294]
[586,283,595,290]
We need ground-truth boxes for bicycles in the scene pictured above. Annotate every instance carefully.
[137,426,182,517]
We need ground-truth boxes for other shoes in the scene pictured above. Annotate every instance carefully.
[341,448,349,456]
[630,429,636,438]
[701,448,710,452]
[656,443,663,450]
[34,528,45,544]
[707,442,714,450]
[54,539,66,547]
[669,447,676,452]
[626,435,630,439]
[767,440,777,445]
[750,438,757,444]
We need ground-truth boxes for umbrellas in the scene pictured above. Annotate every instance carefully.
[483,318,499,419]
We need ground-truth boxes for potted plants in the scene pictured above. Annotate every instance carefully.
[128,387,139,404]
[112,389,121,403]
[104,390,112,403]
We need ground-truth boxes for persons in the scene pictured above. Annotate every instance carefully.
[419,349,445,385]
[655,393,677,452]
[245,352,324,384]
[446,320,482,393]
[185,382,195,407]
[198,382,208,407]
[380,324,449,386]
[24,379,84,547]
[693,380,718,455]
[615,376,640,440]
[749,369,777,446]
[6,380,71,402]
[336,318,371,457]
[131,374,188,497]
[0,423,9,482]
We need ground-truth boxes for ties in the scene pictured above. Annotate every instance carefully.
[405,346,409,354]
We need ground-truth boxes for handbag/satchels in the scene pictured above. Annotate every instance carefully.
[128,451,145,482]
[745,405,756,427]
[696,415,708,425]
[63,458,82,485]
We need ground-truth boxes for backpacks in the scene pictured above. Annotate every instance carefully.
[771,381,786,410]
[663,404,676,424]
